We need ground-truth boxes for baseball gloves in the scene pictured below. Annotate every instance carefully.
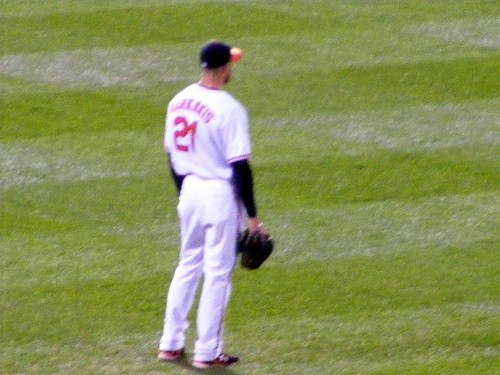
[237,226,277,271]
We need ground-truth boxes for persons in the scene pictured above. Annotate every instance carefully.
[155,40,268,370]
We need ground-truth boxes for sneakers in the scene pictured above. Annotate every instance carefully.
[158,348,185,362]
[191,351,240,368]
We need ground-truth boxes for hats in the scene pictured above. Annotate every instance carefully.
[199,39,243,69]
